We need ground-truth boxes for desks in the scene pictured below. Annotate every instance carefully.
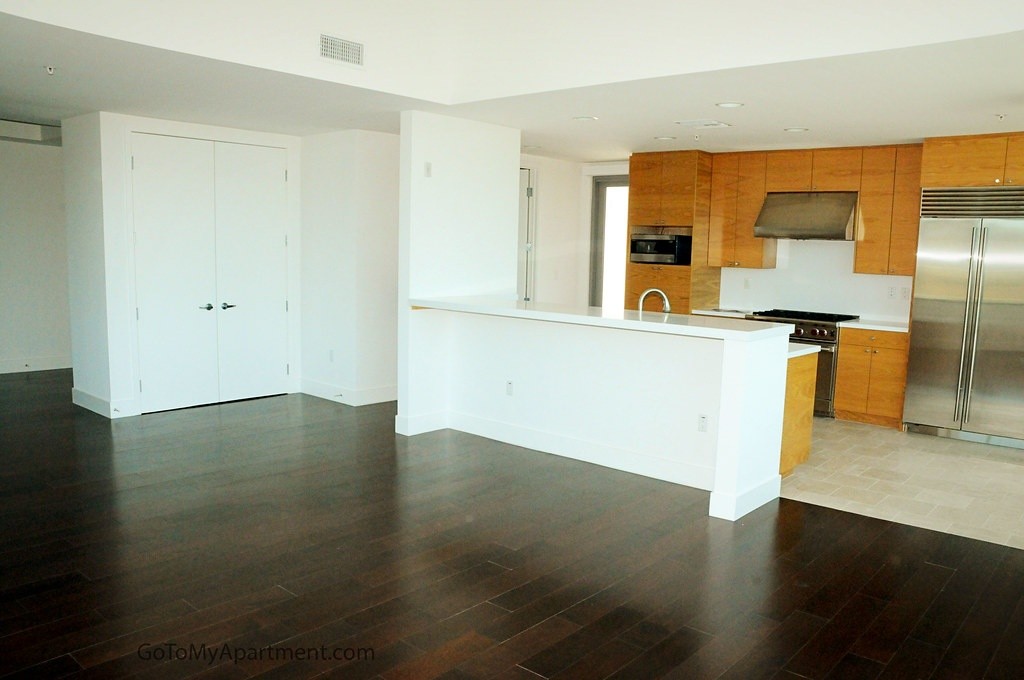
[411,296,794,521]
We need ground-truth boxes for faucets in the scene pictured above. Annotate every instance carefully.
[638,286,672,314]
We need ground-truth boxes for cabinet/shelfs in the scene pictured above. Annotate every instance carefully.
[765,146,862,192]
[625,264,720,315]
[919,132,1024,187]
[834,328,910,431]
[707,151,777,269]
[854,143,923,275]
[631,150,712,227]
[780,342,821,478]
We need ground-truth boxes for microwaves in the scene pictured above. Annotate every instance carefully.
[628,226,692,265]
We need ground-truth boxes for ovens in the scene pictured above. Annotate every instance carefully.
[788,337,838,417]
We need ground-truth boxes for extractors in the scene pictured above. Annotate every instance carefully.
[753,192,857,241]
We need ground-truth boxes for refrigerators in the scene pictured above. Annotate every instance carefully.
[902,186,1024,451]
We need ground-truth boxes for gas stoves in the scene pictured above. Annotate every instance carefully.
[746,309,860,341]
